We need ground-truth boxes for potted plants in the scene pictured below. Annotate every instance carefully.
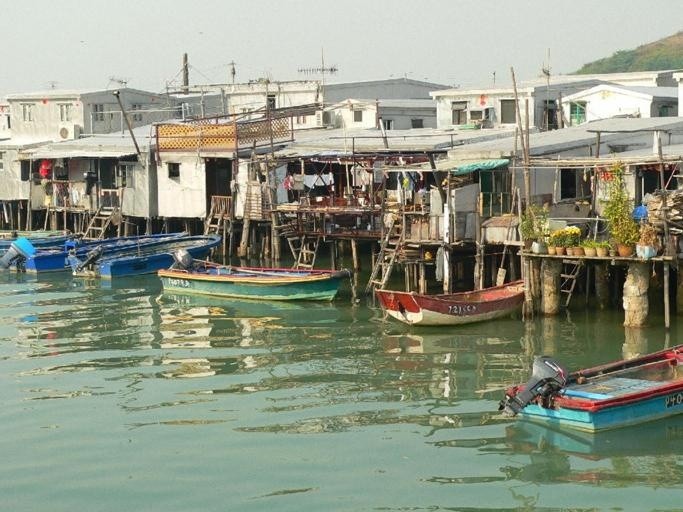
[520,163,661,258]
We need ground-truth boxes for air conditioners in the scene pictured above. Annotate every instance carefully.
[315,110,336,126]
[60,125,79,142]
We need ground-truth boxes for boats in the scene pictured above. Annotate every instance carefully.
[0,229,220,278]
[159,255,350,306]
[498,344,682,431]
[372,278,528,328]
